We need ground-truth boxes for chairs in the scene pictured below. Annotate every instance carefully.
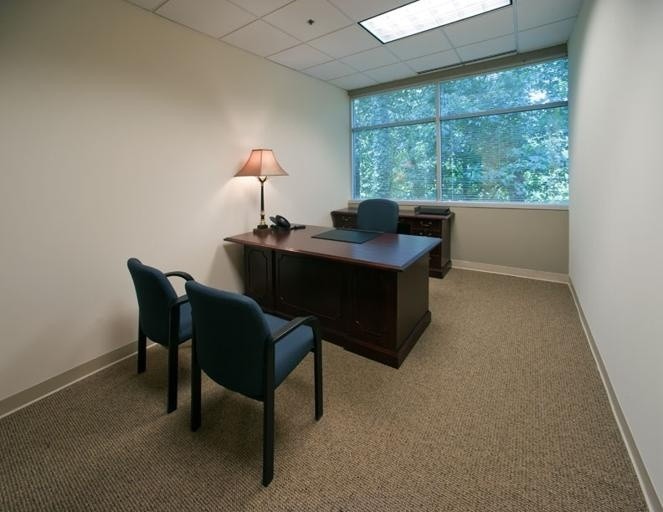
[184,279,325,488]
[126,257,194,415]
[356,198,399,234]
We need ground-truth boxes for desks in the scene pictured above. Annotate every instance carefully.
[222,224,444,370]
[330,206,455,279]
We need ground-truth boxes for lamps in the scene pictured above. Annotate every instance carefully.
[233,148,289,235]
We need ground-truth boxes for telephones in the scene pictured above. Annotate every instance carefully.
[270,215,291,230]
